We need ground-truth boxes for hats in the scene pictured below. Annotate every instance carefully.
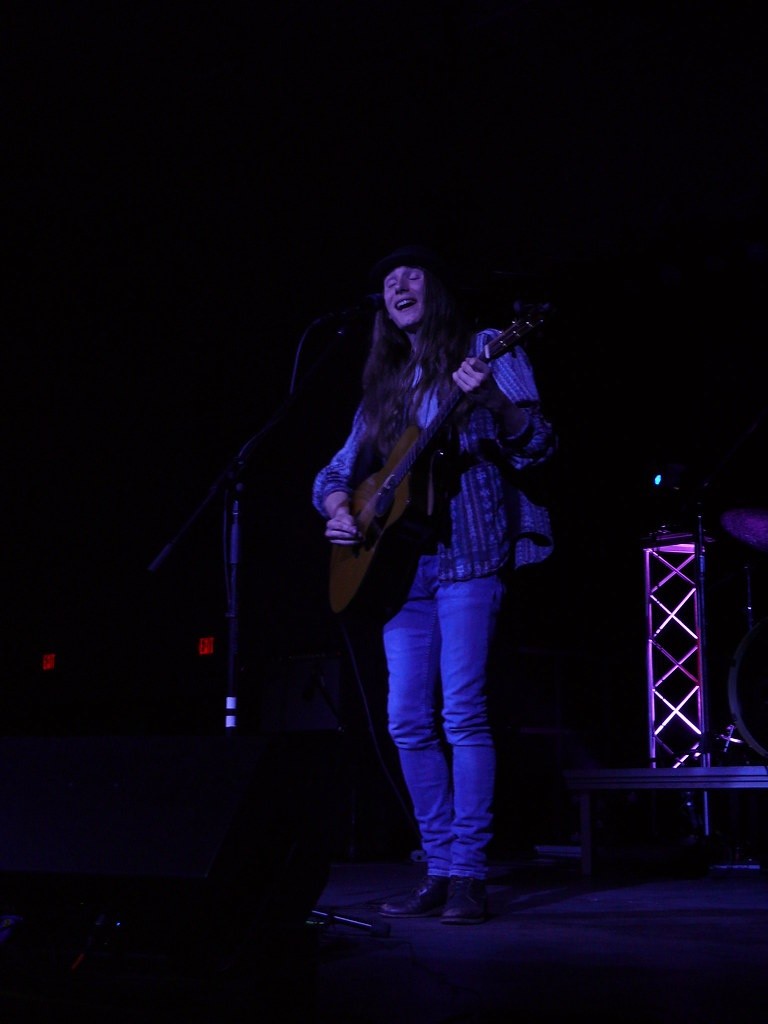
[375,246,429,277]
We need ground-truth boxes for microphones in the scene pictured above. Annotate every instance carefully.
[311,294,384,331]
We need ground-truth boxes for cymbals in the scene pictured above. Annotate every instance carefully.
[720,507,768,553]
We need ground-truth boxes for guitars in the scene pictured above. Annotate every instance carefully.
[326,296,556,624]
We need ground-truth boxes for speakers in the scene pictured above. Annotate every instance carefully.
[0,732,351,931]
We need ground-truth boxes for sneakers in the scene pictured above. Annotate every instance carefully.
[377,875,489,925]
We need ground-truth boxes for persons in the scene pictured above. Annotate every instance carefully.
[313,248,556,922]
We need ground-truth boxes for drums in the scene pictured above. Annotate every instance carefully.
[726,611,768,760]
